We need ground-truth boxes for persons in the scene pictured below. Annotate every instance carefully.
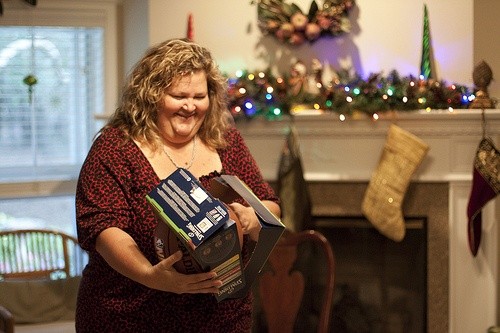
[75,38,282,333]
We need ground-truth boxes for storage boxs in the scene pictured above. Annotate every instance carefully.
[145,168,286,302]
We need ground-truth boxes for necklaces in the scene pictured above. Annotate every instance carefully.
[159,135,196,169]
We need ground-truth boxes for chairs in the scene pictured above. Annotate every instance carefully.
[0,229,87,333]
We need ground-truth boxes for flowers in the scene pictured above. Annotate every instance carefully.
[259,0,355,47]
